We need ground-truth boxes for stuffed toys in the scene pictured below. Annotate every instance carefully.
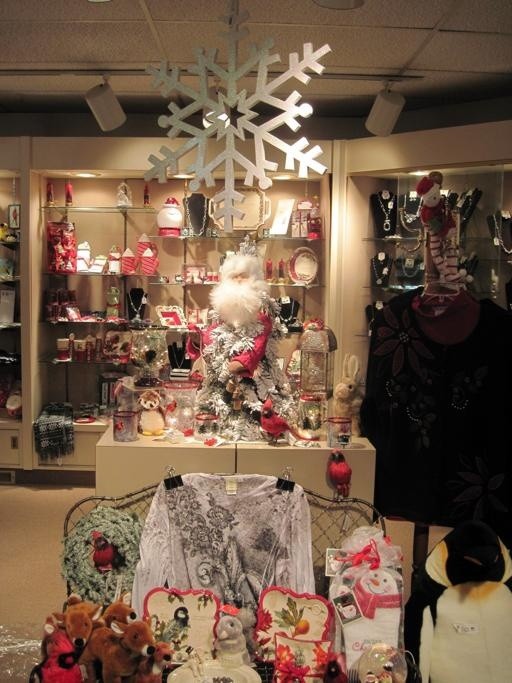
[137,390,162,433]
[332,353,364,439]
[51,587,160,682]
[214,615,254,667]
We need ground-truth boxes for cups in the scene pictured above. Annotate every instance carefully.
[107,241,160,276]
[112,410,140,443]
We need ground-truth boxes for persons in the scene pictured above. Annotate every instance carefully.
[275,295,301,326]
[168,341,192,372]
[197,244,279,428]
[182,191,213,237]
[126,286,149,321]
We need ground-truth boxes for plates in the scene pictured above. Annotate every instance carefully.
[250,585,334,666]
[270,632,334,683]
[140,587,222,670]
[283,349,302,379]
[277,356,285,371]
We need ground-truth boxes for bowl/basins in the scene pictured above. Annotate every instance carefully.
[286,246,319,285]
[166,658,262,683]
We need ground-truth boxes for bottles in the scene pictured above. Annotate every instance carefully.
[161,380,200,434]
[278,258,285,278]
[47,182,54,206]
[207,272,212,281]
[194,412,221,441]
[327,417,353,449]
[56,331,105,362]
[298,395,321,432]
[265,258,272,280]
[65,181,73,207]
[301,329,330,392]
[212,272,219,282]
[143,185,150,207]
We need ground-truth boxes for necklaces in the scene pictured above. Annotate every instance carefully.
[128,291,147,319]
[371,185,511,292]
[186,196,207,235]
[173,342,187,368]
[278,300,295,322]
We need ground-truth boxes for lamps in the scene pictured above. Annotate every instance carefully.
[363,85,407,138]
[84,73,128,133]
[200,74,233,128]
